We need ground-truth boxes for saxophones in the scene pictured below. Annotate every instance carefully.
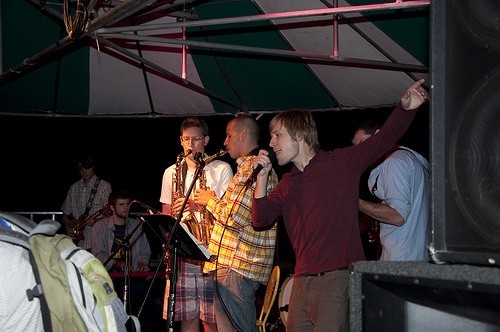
[165,149,187,218]
[182,148,228,260]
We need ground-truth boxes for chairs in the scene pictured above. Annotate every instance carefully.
[256,266,281,332]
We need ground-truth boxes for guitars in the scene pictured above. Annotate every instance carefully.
[61,203,112,247]
[356,209,383,261]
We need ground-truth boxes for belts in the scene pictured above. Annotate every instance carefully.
[301,266,350,277]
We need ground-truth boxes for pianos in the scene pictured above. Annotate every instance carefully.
[107,269,167,287]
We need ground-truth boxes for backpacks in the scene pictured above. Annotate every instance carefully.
[0,212,140,332]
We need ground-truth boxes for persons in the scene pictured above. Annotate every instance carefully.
[91,190,151,272]
[61,157,112,252]
[251,79,430,332]
[160,119,234,332]
[345,121,431,262]
[0,216,52,332]
[194,115,278,332]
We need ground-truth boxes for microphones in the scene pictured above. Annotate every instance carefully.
[244,150,276,185]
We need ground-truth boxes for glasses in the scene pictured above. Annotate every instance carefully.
[182,136,204,142]
